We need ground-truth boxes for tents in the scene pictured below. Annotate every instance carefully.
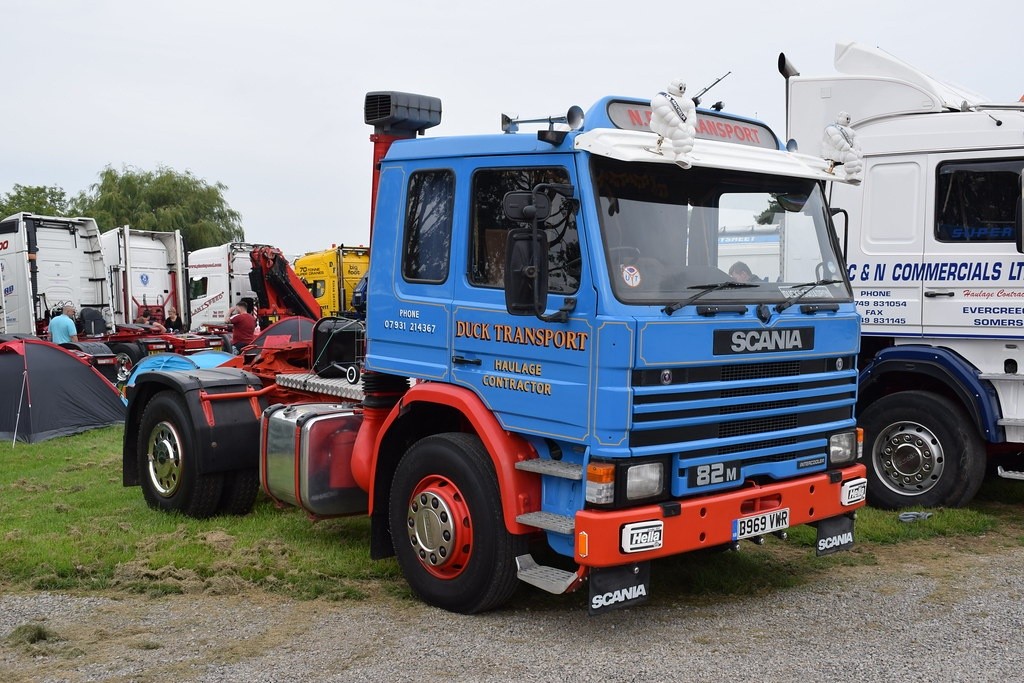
[241,317,324,361]
[0,339,250,447]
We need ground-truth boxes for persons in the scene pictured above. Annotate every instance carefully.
[133,306,185,334]
[224,300,257,355]
[822,111,865,184]
[47,305,79,344]
[729,261,763,283]
[649,78,698,169]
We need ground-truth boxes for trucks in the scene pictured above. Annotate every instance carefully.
[123,90,865,617]
[0,211,369,384]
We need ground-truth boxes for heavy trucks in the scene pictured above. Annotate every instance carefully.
[687,43,1024,511]
